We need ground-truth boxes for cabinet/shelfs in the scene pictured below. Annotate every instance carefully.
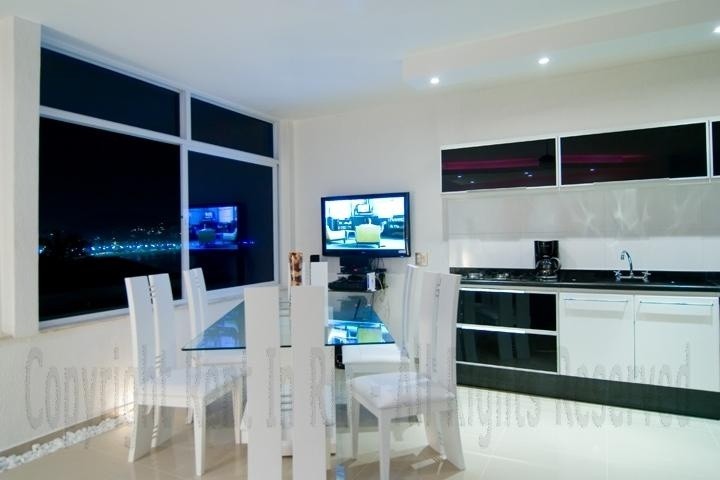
[556,287,720,421]
[456,283,559,398]
[439,115,720,194]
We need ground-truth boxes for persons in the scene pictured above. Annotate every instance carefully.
[353,217,385,235]
[193,222,217,237]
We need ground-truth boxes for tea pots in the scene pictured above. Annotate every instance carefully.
[534,253,561,279]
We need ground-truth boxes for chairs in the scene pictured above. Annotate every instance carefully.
[122,264,467,480]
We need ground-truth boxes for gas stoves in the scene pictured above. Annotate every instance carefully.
[450,268,532,284]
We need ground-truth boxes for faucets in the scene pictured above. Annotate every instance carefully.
[613,249,651,283]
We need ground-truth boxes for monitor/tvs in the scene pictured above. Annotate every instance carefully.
[320,192,410,258]
